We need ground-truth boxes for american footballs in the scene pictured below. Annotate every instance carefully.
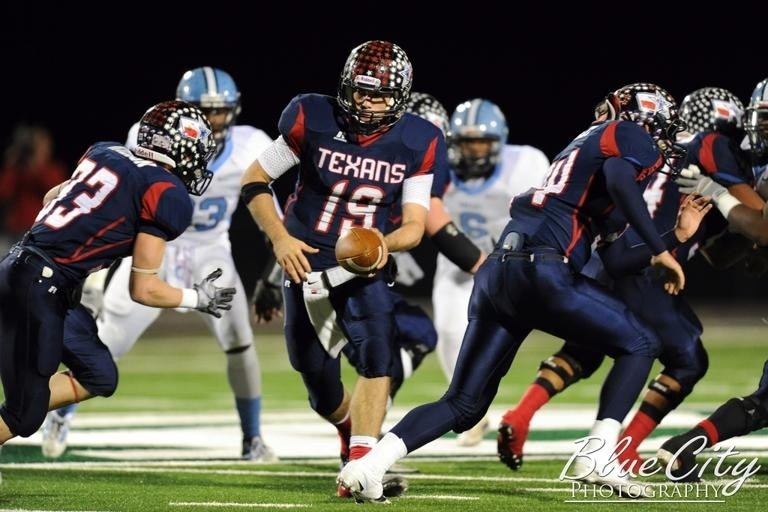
[336,228,384,274]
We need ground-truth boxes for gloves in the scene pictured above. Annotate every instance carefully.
[253,282,283,323]
[193,268,235,319]
[676,166,740,220]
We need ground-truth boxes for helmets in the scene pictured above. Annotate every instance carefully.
[450,99,508,181]
[679,88,745,146]
[134,101,215,196]
[594,83,687,177]
[176,67,240,144]
[337,41,413,134]
[743,81,768,164]
[409,93,450,142]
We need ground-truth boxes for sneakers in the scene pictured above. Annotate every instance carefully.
[498,411,528,469]
[242,437,276,463]
[574,449,652,497]
[41,411,72,457]
[618,454,665,474]
[656,435,701,482]
[337,459,391,503]
[337,473,408,496]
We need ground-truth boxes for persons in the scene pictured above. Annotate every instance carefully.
[0,39,767,505]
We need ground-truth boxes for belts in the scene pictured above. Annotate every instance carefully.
[10,244,79,289]
[486,254,569,262]
